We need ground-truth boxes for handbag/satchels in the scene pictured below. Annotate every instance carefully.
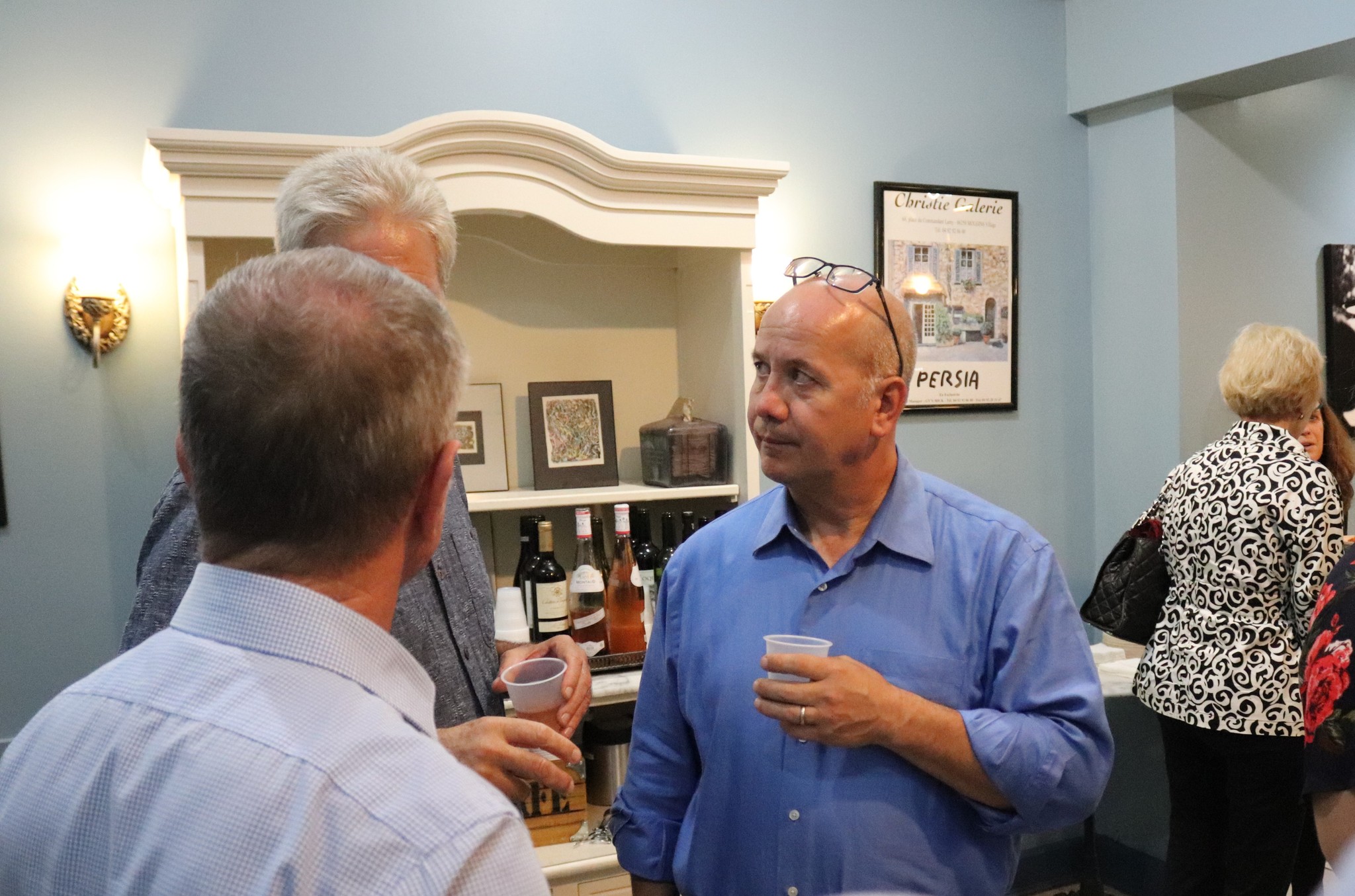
[1079,482,1169,648]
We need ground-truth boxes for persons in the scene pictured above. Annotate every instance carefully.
[1132,322,1355,896]
[1,246,553,896]
[609,258,1113,895]
[119,146,592,801]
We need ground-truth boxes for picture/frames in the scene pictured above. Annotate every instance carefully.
[873,179,1019,415]
[527,379,619,490]
[454,383,509,492]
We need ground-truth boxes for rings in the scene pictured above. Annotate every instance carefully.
[798,705,806,726]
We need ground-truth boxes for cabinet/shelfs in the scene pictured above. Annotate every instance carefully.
[146,103,791,896]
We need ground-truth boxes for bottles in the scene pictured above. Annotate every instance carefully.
[513,514,571,644]
[698,518,709,529]
[568,508,610,657]
[654,512,679,594]
[630,633,632,635]
[591,516,610,595]
[606,504,647,654]
[629,505,661,649]
[682,511,696,543]
[715,510,727,519]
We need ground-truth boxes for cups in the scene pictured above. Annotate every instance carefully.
[500,657,567,734]
[494,587,530,644]
[763,635,833,682]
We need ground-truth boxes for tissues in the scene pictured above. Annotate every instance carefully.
[636,394,732,489]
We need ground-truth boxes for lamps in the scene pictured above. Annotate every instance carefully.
[755,301,773,331]
[65,279,131,368]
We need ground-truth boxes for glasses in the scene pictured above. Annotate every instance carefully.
[1313,398,1324,414]
[783,257,905,378]
